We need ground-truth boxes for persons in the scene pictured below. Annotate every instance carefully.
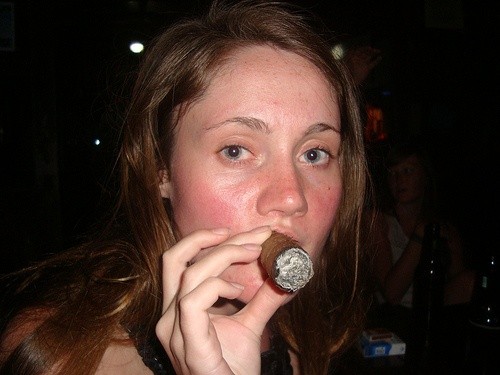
[340,44,384,86]
[358,135,472,375]
[0,0,373,375]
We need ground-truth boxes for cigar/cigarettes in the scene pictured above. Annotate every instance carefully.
[258,230,314,295]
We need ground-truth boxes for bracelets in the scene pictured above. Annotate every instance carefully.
[410,231,424,246]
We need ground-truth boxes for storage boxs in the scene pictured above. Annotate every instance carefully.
[356,328,407,360]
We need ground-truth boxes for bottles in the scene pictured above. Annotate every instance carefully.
[412,219,457,375]
[464,253,500,375]
